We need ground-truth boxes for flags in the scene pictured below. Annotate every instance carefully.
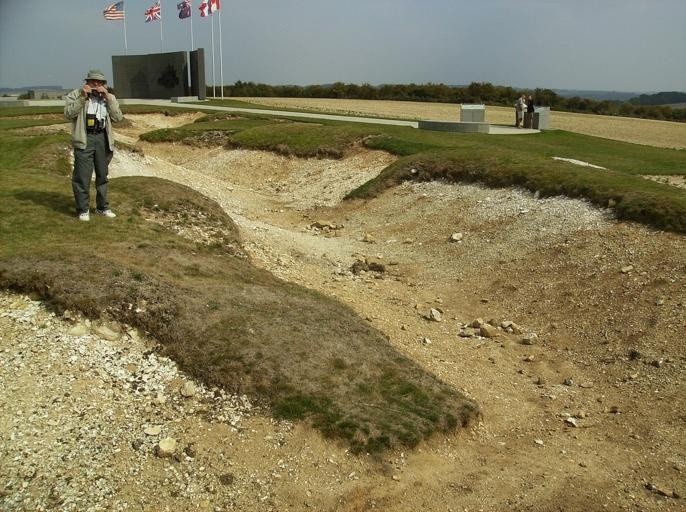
[103,1,123,20]
[176,1,190,20]
[198,0,212,17]
[210,0,220,12]
[144,2,161,24]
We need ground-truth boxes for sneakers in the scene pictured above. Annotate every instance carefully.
[96,206,116,218]
[78,210,90,221]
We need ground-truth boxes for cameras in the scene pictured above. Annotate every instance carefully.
[87,88,103,100]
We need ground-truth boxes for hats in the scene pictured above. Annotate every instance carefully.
[83,68,107,83]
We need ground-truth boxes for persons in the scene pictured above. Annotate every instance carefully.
[514,93,529,129]
[523,95,534,129]
[63,69,123,222]
